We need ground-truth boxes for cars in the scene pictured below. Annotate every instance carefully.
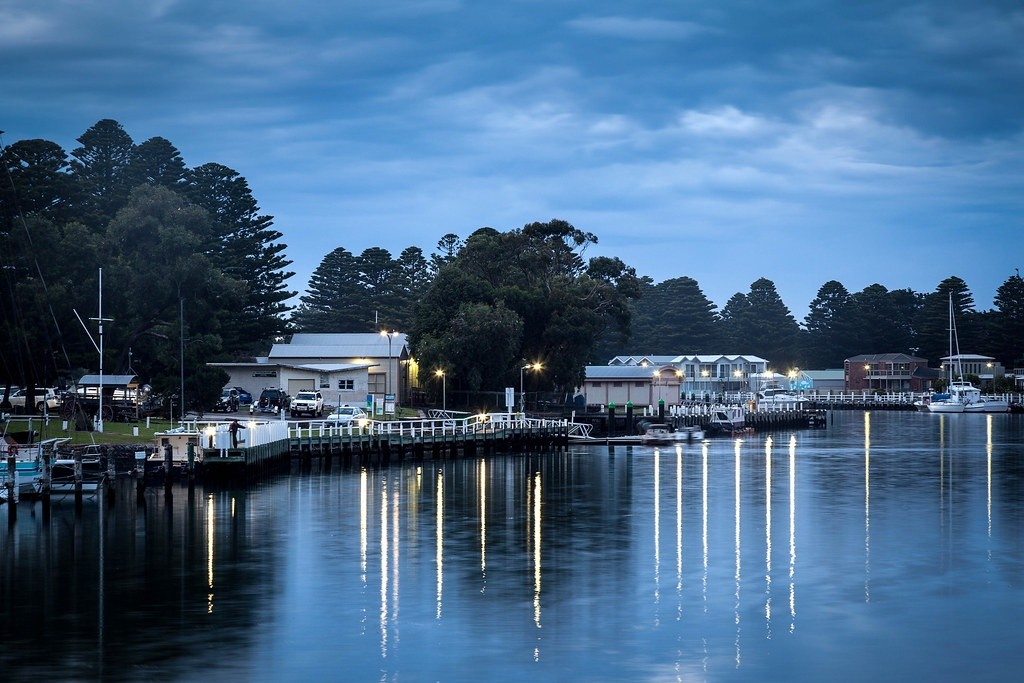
[328,407,366,428]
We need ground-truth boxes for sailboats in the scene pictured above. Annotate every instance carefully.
[0,130,110,502]
[947,290,1009,411]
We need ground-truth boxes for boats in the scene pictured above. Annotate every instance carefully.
[674,425,706,443]
[643,423,672,444]
[927,399,964,413]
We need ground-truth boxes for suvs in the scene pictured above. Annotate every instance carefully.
[290,389,324,416]
[260,387,291,411]
[215,388,239,412]
[9,386,61,412]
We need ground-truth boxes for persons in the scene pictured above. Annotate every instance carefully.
[229,419,245,448]
[8,442,18,474]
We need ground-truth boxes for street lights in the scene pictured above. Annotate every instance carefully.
[381,329,398,422]
[702,371,708,405]
[677,370,682,403]
[768,371,775,409]
[655,370,662,400]
[520,364,541,417]
[436,371,445,417]
[737,370,743,410]
[792,371,799,411]
[866,365,871,395]
